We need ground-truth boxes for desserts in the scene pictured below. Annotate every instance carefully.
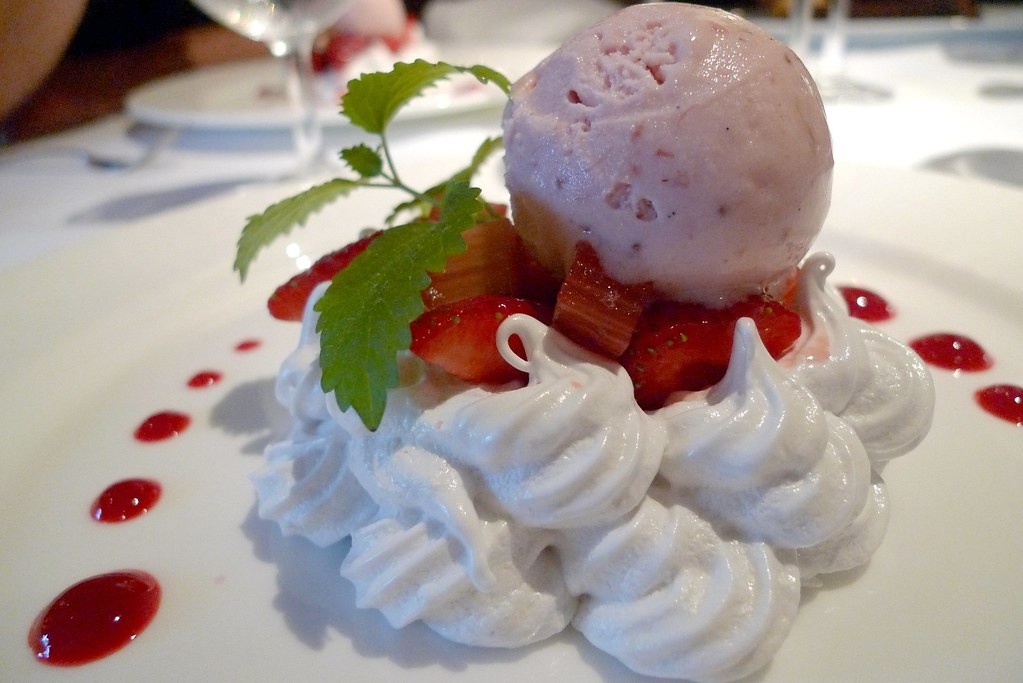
[288,0,438,103]
[245,0,936,682]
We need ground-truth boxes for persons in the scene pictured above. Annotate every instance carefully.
[0,0,426,158]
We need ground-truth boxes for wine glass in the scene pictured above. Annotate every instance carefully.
[196,0,356,175]
[783,0,896,102]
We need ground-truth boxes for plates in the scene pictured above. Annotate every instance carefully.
[0,239,1023,683]
[121,47,546,130]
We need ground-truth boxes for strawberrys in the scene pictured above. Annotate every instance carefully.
[264,198,803,405]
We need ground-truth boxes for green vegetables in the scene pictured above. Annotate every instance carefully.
[233,58,519,432]
